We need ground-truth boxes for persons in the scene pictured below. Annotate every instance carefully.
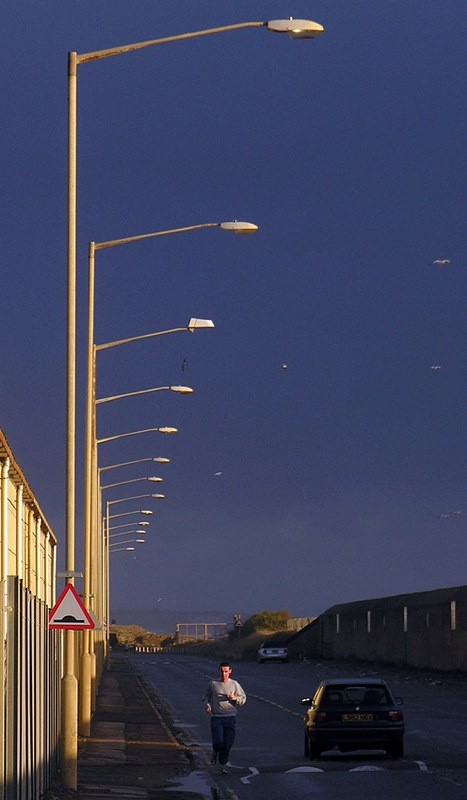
[201,662,247,774]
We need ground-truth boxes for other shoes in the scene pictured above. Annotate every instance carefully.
[220,766,228,774]
[212,754,217,766]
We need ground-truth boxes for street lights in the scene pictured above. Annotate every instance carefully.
[60,17,330,790]
[80,220,257,741]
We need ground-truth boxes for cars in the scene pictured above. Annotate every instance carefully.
[300,679,405,761]
[258,641,290,663]
